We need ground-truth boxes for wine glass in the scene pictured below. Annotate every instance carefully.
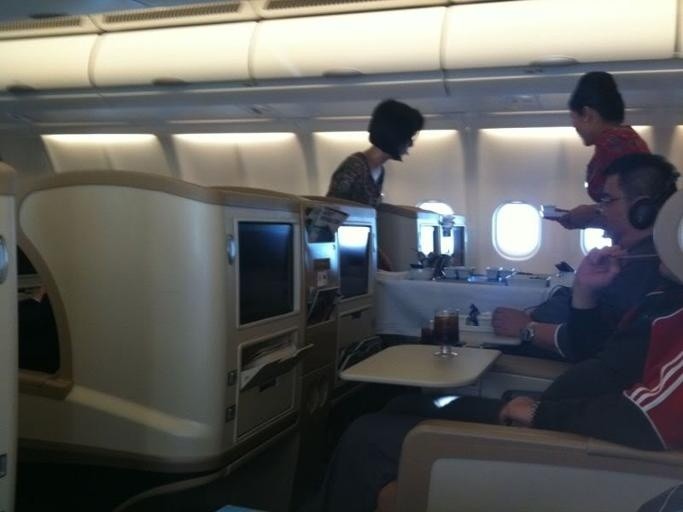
[420,252,452,281]
[419,307,465,361]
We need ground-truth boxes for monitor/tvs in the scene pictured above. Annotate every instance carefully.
[440,225,466,267]
[421,224,440,258]
[234,216,303,327]
[335,222,374,302]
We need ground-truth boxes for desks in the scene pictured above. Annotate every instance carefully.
[460,332,523,347]
[339,344,503,395]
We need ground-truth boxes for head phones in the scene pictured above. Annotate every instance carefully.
[629,170,682,229]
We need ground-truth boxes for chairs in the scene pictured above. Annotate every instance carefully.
[303,196,377,416]
[17,170,306,511]
[398,355,683,512]
[0,162,17,512]
[380,205,469,270]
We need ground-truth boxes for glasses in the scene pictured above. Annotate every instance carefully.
[596,192,629,206]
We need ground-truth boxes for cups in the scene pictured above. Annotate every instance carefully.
[444,265,478,279]
[557,269,575,286]
